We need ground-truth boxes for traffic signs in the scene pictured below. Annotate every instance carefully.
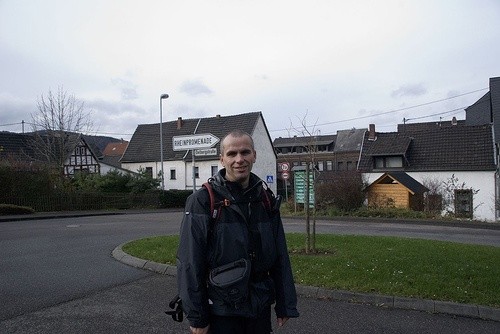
[172,133,220,151]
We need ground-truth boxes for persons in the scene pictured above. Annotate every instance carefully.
[175,129,299,334]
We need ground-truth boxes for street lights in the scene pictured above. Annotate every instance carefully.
[160,94,169,190]
